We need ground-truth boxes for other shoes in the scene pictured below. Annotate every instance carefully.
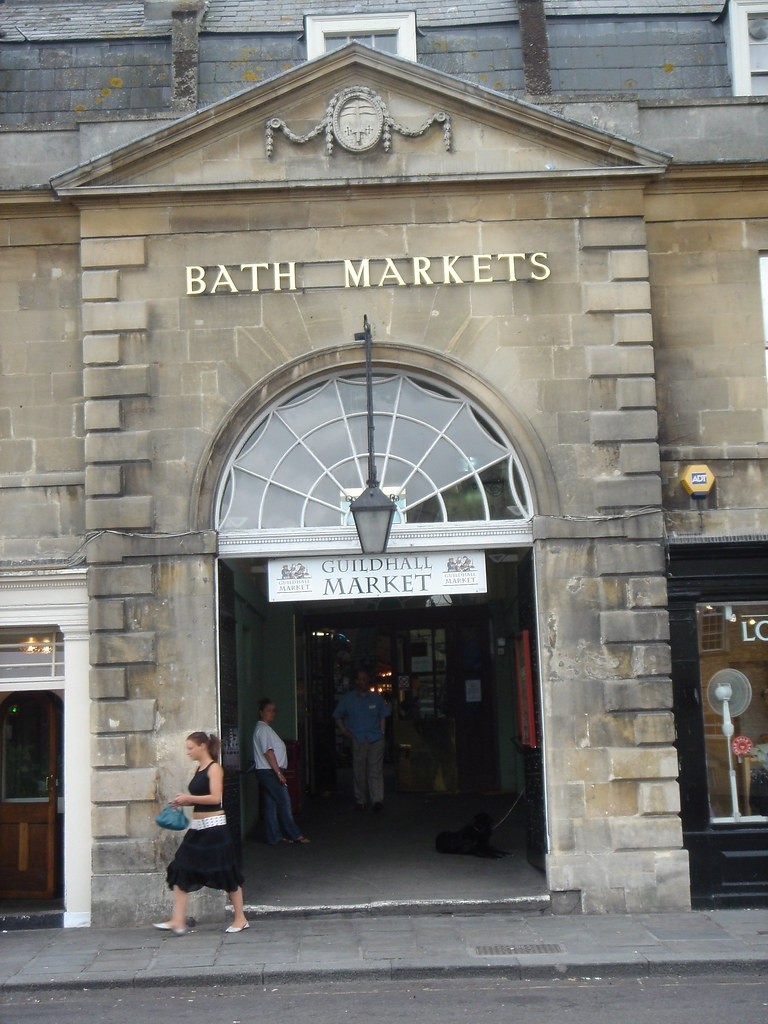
[355,804,367,811]
[293,836,311,844]
[154,923,186,936]
[225,920,250,933]
[373,802,383,812]
[278,838,293,845]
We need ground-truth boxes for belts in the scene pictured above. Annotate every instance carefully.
[189,815,227,830]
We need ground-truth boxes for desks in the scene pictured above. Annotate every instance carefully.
[739,756,760,815]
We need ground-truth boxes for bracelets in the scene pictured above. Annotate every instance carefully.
[278,770,282,775]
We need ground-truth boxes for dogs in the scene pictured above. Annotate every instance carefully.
[434,811,515,861]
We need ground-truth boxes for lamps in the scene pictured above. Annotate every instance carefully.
[349,314,397,555]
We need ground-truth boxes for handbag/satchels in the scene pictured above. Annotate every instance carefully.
[155,803,189,830]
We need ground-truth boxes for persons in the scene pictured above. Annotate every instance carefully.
[331,668,392,810]
[153,733,249,935]
[252,700,314,848]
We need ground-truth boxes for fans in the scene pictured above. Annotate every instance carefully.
[707,668,768,823]
